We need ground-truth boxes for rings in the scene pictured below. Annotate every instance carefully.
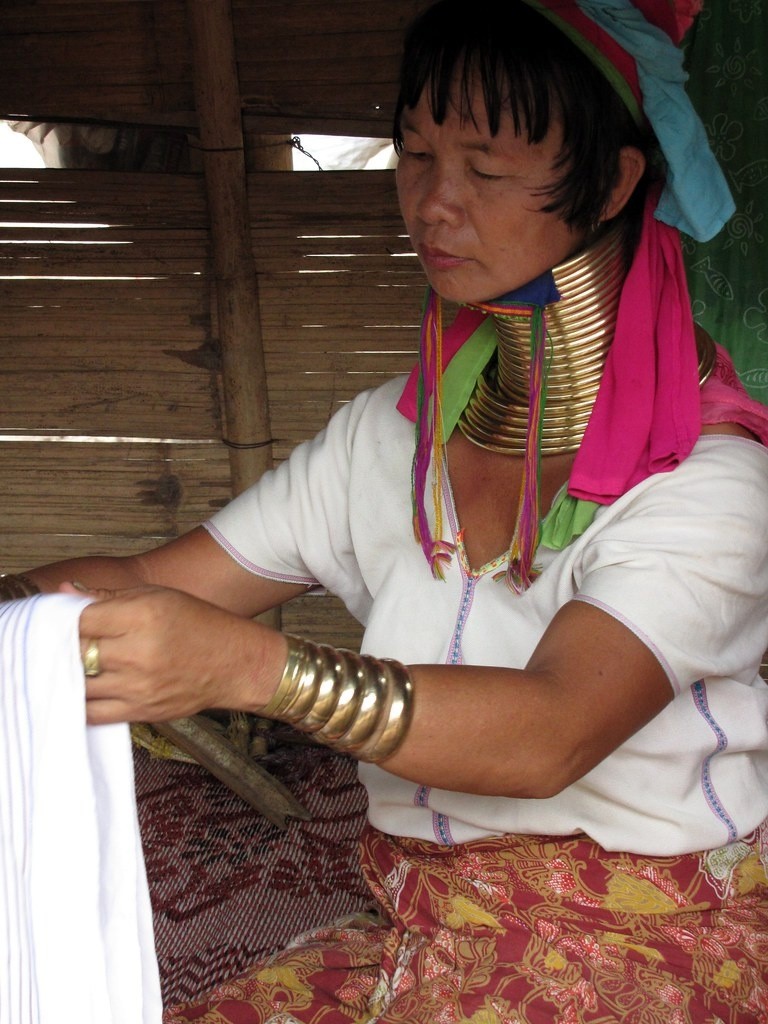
[82,639,100,678]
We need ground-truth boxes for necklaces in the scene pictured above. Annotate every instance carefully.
[459,223,722,457]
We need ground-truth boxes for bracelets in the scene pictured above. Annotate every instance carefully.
[250,630,414,762]
[1,574,41,604]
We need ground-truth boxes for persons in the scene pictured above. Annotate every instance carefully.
[1,0,768,1022]
